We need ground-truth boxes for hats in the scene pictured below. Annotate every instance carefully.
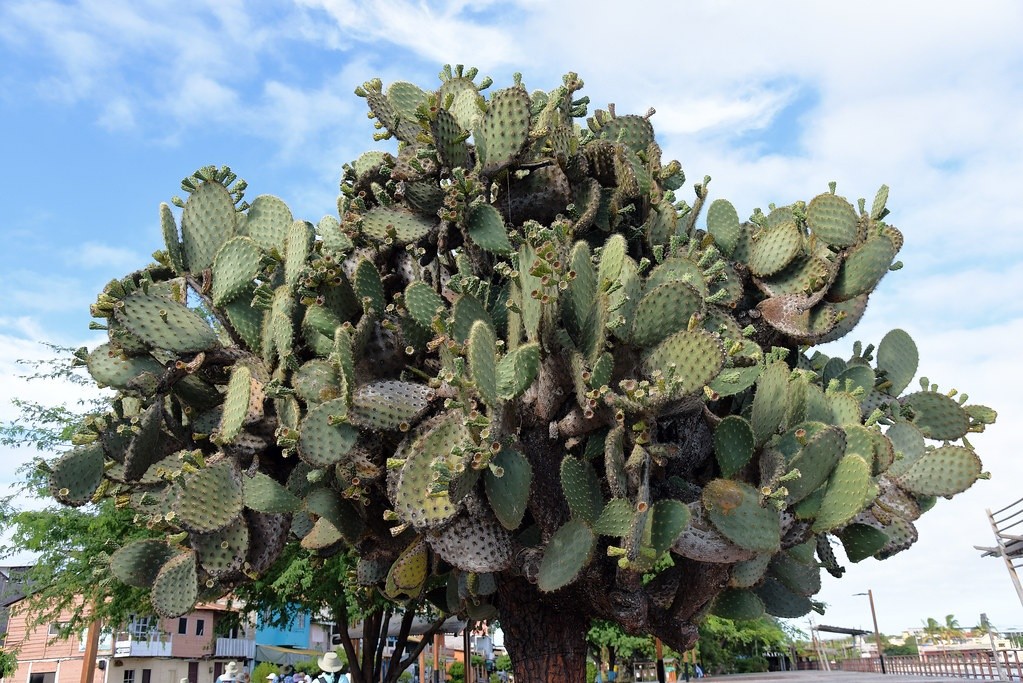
[237,672,250,683]
[280,663,292,674]
[220,674,233,681]
[225,662,238,673]
[318,652,343,672]
[180,678,189,683]
[266,673,276,679]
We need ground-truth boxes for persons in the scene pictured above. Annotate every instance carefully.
[596,663,618,683]
[180,678,190,683]
[235,672,250,683]
[216,661,239,683]
[272,667,311,683]
[497,669,505,683]
[311,651,350,683]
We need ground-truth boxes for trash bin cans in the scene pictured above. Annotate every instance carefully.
[765,655,785,672]
[665,667,676,683]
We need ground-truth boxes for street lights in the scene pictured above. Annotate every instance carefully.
[852,589,886,674]
